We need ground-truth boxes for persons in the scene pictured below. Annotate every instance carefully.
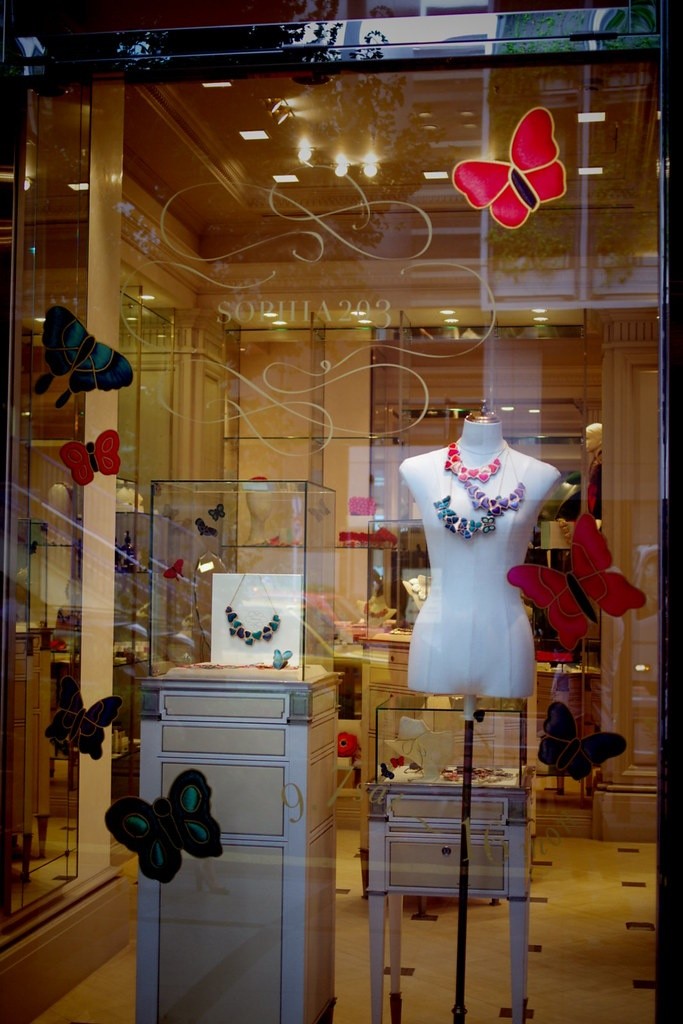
[397,412,565,699]
[245,477,274,545]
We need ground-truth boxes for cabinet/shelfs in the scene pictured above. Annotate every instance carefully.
[355,633,539,902]
[366,763,535,1024]
[219,310,414,632]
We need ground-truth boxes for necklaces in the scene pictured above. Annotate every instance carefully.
[226,573,281,646]
[446,439,505,483]
[464,449,527,516]
[432,438,506,542]
